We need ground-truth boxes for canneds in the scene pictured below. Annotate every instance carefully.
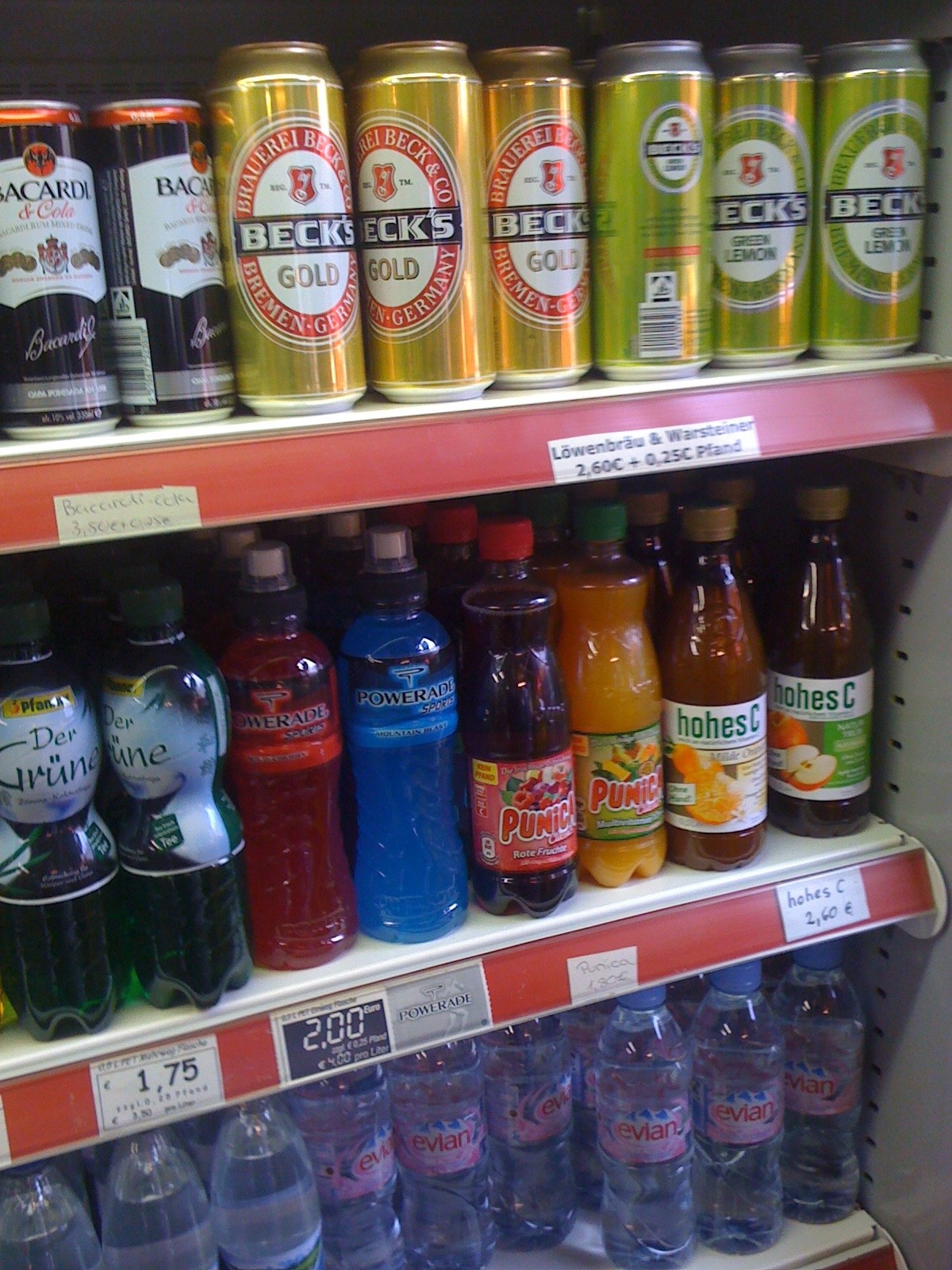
[192,38,594,418]
[0,96,235,442]
[566,37,927,382]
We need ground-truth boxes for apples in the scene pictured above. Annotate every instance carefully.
[764,713,836,790]
[512,765,572,810]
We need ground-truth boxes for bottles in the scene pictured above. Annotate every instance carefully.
[0,472,878,1041]
[0,936,860,1270]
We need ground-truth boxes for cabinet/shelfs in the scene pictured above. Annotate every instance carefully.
[3,1,950,1267]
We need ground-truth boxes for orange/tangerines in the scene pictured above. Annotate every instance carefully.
[671,744,741,824]
